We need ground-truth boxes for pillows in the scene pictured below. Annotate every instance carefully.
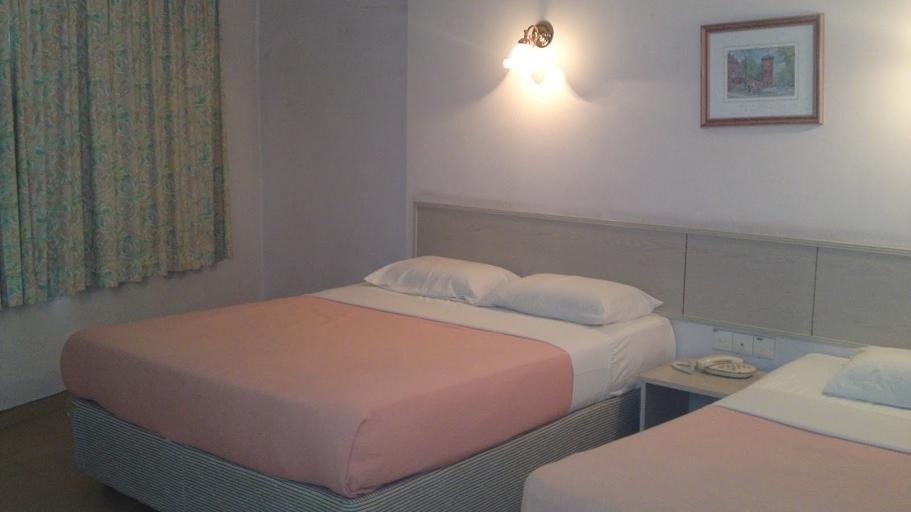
[487,271,664,326]
[365,255,520,308]
[822,344,911,408]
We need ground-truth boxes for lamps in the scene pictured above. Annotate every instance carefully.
[502,19,553,74]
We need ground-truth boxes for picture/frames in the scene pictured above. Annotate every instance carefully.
[699,12,824,127]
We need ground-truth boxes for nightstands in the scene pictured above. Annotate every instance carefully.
[638,357,769,433]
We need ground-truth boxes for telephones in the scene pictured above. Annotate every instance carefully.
[695,355,757,378]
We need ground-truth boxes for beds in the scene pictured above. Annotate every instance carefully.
[521,227,911,512]
[61,200,689,512]
